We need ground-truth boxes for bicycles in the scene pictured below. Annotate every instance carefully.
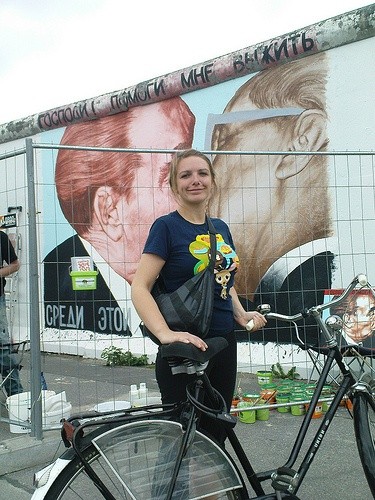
[30,273,375,500]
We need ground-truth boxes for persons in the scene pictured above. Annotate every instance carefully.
[131,150,268,500]
[0,232,24,397]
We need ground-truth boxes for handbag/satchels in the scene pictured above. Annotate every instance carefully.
[147,216,217,345]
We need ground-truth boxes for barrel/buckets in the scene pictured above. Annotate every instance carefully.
[230,370,353,424]
[69,263,99,290]
[132,396,163,412]
[94,401,132,420]
[5,390,72,434]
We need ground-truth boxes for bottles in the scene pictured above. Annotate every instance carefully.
[128,385,139,402]
[138,383,148,399]
